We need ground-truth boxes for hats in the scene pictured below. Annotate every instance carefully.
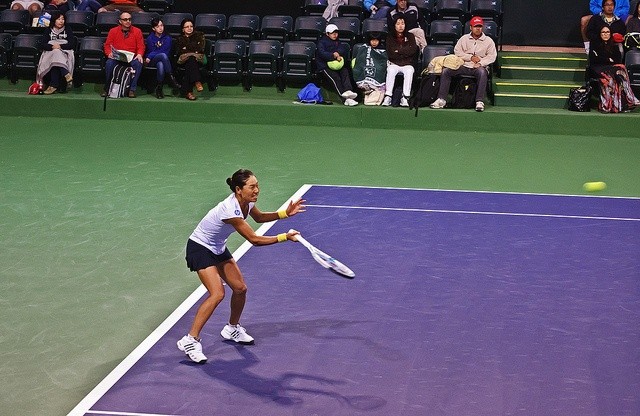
[326,24,339,33]
[469,16,484,27]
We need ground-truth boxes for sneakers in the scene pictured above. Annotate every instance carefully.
[400,98,409,107]
[430,98,447,109]
[382,96,393,106]
[342,91,358,99]
[44,87,57,94]
[475,101,485,112]
[64,73,73,83]
[177,336,209,364]
[344,99,359,106]
[186,92,196,100]
[195,82,204,92]
[221,325,256,345]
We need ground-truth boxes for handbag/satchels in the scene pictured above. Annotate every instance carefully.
[27,82,43,96]
[568,85,592,112]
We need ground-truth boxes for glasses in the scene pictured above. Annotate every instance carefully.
[601,31,611,34]
[183,26,194,28]
[120,18,132,21]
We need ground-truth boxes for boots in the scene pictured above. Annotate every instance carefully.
[168,74,182,89]
[156,90,164,99]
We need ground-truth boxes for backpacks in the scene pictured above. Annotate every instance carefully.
[103,63,137,112]
[410,74,442,118]
[452,79,478,110]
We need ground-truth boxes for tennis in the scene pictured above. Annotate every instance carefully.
[584,182,606,191]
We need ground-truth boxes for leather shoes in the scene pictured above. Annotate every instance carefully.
[101,91,107,97]
[129,91,136,98]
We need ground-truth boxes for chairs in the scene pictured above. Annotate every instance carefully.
[0,0,11,11]
[397,50,422,106]
[312,43,349,90]
[77,35,106,88]
[584,65,603,113]
[245,40,282,93]
[360,18,387,48]
[419,45,454,107]
[222,15,261,43]
[93,12,121,36]
[282,40,315,94]
[259,15,294,41]
[129,13,160,40]
[139,1,172,15]
[303,1,328,17]
[409,1,436,21]
[213,39,247,92]
[435,1,468,22]
[338,0,363,22]
[453,63,495,109]
[163,12,193,37]
[352,43,364,60]
[462,20,500,77]
[0,33,12,81]
[66,10,94,38]
[470,1,503,51]
[144,39,157,94]
[330,16,360,48]
[623,49,639,89]
[24,11,53,35]
[0,9,29,36]
[423,20,431,46]
[195,14,227,43]
[428,20,463,46]
[173,39,212,92]
[292,17,325,44]
[12,34,45,86]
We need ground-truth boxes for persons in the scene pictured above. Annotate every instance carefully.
[176,168,307,362]
[431,16,497,112]
[587,0,627,41]
[171,17,208,101]
[317,24,360,107]
[389,0,422,29]
[362,0,398,20]
[9,0,44,12]
[37,13,74,95]
[626,0,640,32]
[98,0,146,14]
[356,30,387,89]
[580,0,631,57]
[101,12,146,99]
[589,26,640,113]
[146,17,182,98]
[382,17,417,107]
